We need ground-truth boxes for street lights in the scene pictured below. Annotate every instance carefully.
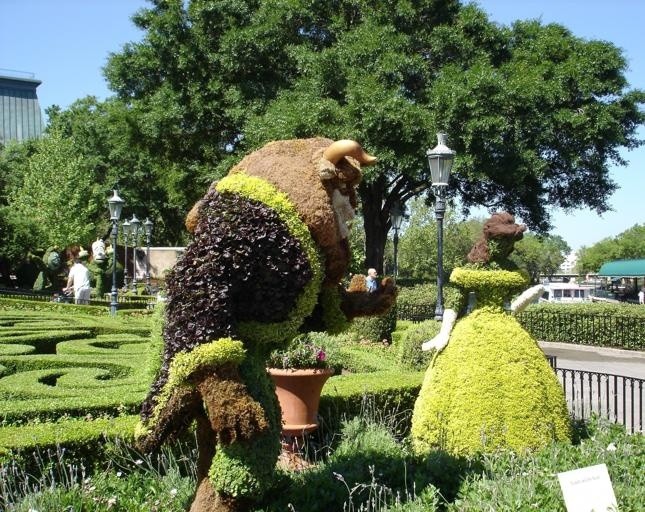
[390,200,403,284]
[109,190,154,315]
[426,132,456,319]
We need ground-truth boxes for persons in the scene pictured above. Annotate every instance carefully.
[638,288,645,305]
[366,268,377,292]
[62,258,91,305]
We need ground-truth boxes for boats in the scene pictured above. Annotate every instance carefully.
[538,259,645,302]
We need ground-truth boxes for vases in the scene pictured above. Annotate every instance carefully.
[265,364,330,436]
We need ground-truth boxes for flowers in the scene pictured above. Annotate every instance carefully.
[262,336,330,371]
